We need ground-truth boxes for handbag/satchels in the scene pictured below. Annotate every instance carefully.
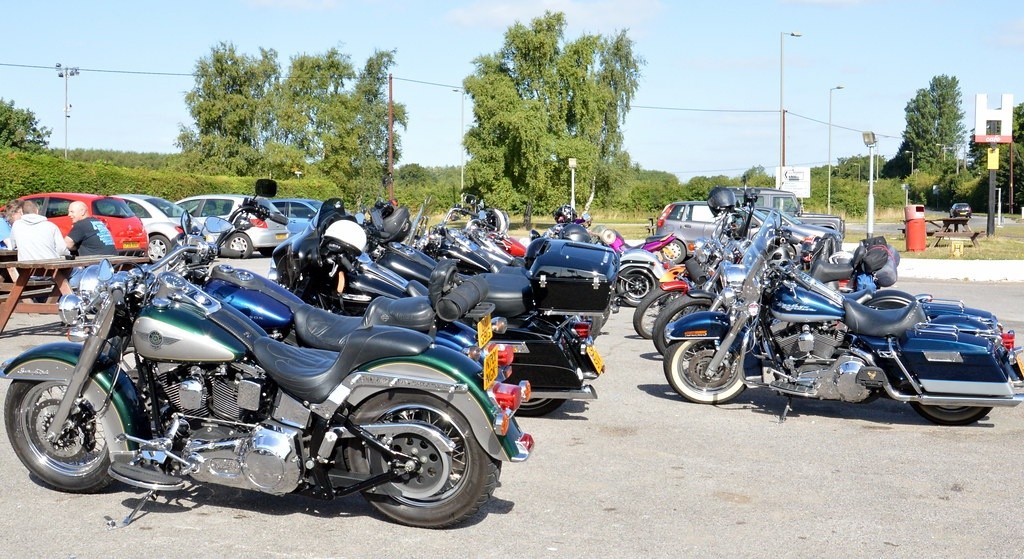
[854,236,900,292]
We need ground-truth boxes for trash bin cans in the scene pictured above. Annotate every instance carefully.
[904,205,927,251]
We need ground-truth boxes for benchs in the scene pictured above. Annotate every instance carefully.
[0,280,79,299]
[926,230,986,239]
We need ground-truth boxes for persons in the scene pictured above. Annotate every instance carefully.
[64,201,118,285]
[0,200,23,250]
[11,200,70,261]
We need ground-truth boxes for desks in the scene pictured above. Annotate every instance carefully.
[0,253,151,334]
[900,217,982,249]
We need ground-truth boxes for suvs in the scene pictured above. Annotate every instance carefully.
[725,186,847,241]
[657,200,842,267]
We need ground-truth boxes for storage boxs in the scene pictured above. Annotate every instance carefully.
[903,338,1014,396]
[524,238,620,312]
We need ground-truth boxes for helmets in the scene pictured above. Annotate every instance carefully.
[323,220,367,255]
[555,205,578,224]
[707,188,736,216]
[374,206,411,245]
[558,224,590,244]
[484,207,509,238]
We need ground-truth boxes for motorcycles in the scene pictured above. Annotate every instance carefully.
[122,179,901,433]
[0,215,535,529]
[663,208,1024,427]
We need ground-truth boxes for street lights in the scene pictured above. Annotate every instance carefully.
[452,89,463,208]
[995,188,1002,225]
[936,143,945,161]
[827,86,844,215]
[568,157,576,210]
[862,130,877,240]
[905,151,914,174]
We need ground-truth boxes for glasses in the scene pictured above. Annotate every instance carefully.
[13,210,23,216]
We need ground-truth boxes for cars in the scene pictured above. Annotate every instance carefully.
[110,193,199,265]
[950,203,972,218]
[164,192,293,259]
[0,191,149,256]
[270,197,324,237]
